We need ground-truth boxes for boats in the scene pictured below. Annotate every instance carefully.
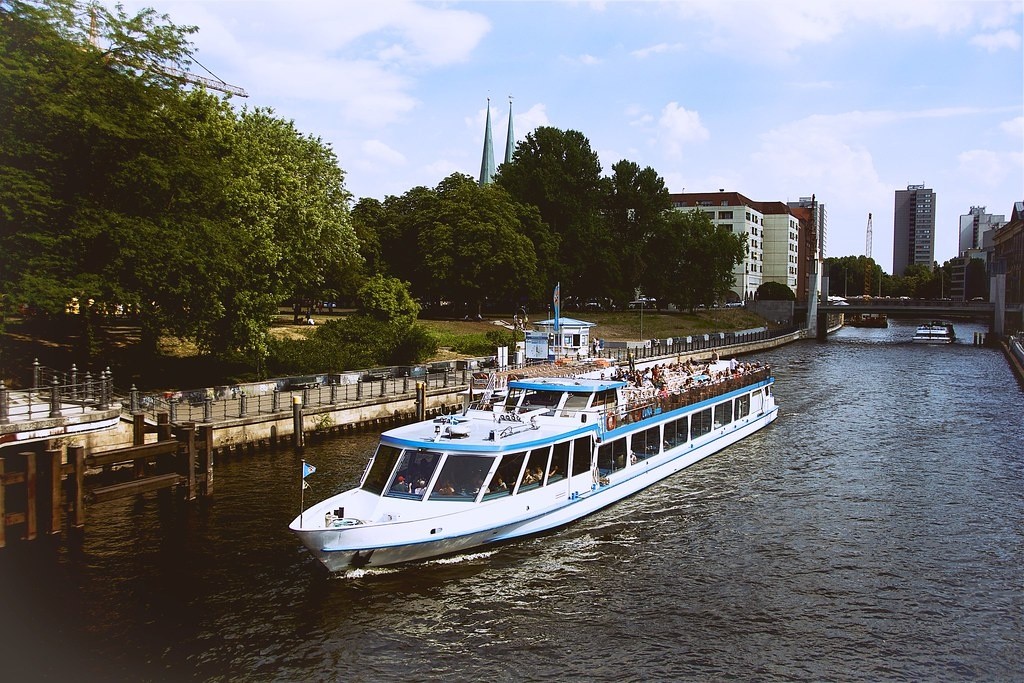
[288,282,779,572]
[912,320,956,344]
[850,314,888,328]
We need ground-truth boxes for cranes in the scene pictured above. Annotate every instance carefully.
[73,7,249,98]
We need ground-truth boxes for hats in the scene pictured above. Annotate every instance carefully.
[398,476,405,481]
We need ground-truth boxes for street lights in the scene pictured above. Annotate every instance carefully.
[513,308,527,350]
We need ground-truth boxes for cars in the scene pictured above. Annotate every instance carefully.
[584,297,745,308]
[856,296,984,301]
[833,301,849,306]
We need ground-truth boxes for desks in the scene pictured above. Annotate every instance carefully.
[596,427,707,476]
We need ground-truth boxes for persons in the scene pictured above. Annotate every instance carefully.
[315,301,322,315]
[434,478,461,495]
[465,314,469,321]
[507,473,516,495]
[518,316,524,328]
[664,440,669,446]
[491,478,508,494]
[477,313,482,321]
[473,478,490,494]
[600,348,764,413]
[304,311,310,325]
[592,334,602,354]
[513,313,518,329]
[391,474,432,497]
[523,312,529,328]
[304,300,315,313]
[646,443,653,453]
[522,463,558,487]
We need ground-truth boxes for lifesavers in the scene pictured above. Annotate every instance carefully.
[632,404,641,422]
[607,411,615,431]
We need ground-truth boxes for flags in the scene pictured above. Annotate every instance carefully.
[304,462,316,479]
[303,480,313,492]
